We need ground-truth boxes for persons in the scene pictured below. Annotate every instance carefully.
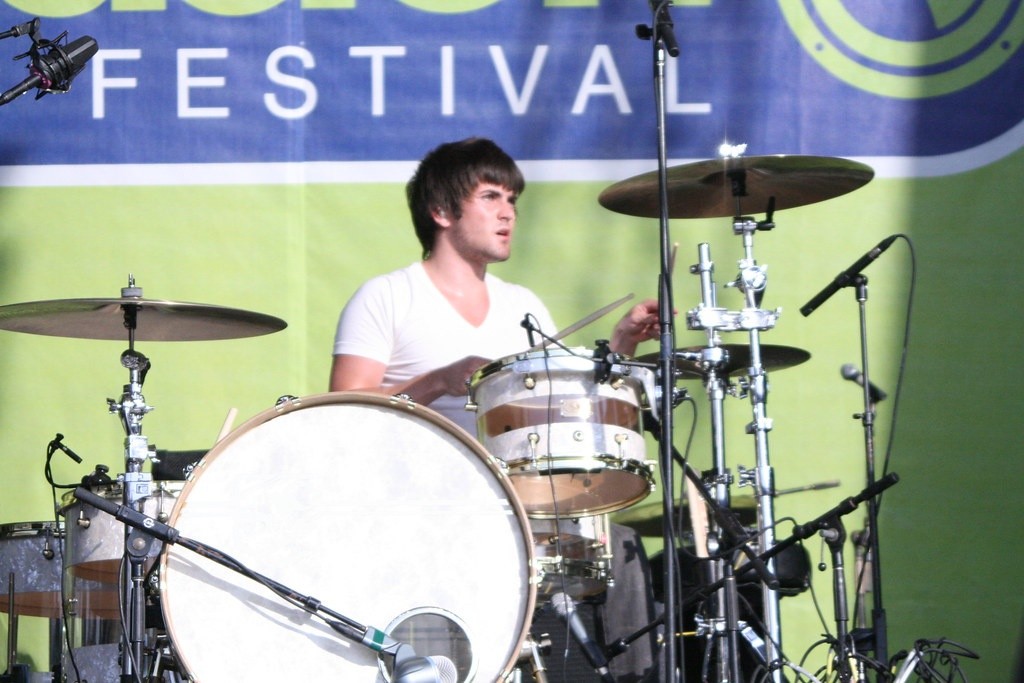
[331,136,679,683]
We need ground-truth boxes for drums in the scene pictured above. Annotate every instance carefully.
[524,509,614,600]
[464,345,658,521]
[57,481,182,586]
[150,390,545,683]
[0,520,124,619]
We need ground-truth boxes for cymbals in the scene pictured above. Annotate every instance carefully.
[0,297,290,342]
[595,155,875,221]
[624,343,813,377]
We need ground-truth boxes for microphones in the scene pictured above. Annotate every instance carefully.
[641,395,685,431]
[53,440,82,463]
[0,35,98,106]
[525,314,535,347]
[327,618,458,683]
[840,363,886,401]
[800,234,899,318]
[551,591,616,683]
